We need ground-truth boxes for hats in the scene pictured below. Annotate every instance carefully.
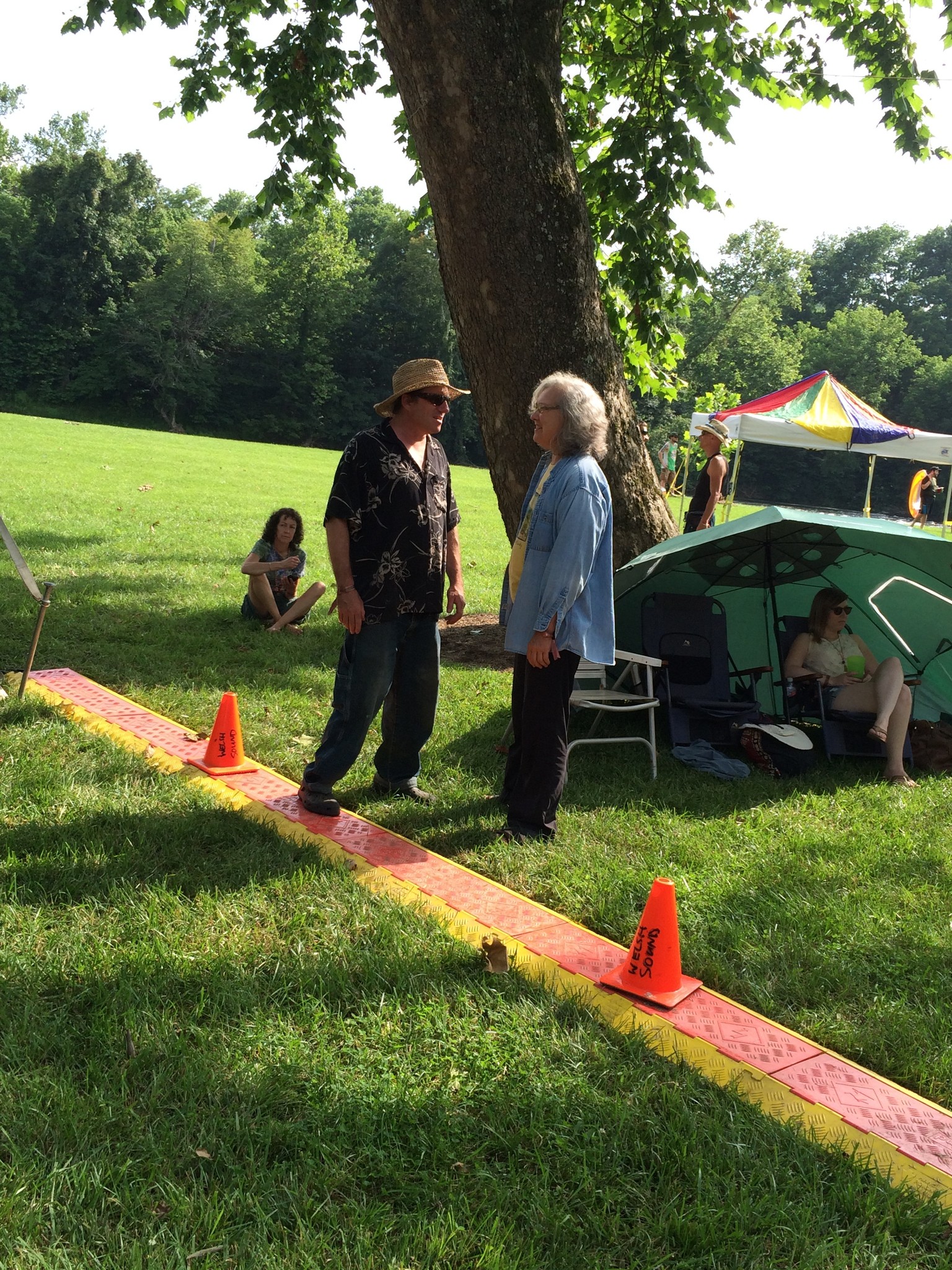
[930,466,942,471]
[695,419,733,448]
[374,358,472,418]
[668,432,680,439]
[731,723,813,750]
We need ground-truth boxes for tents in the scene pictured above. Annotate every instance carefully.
[679,370,952,538]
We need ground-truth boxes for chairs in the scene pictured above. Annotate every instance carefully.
[565,657,660,781]
[639,592,774,748]
[773,616,921,769]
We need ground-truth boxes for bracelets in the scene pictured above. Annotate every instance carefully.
[822,675,830,688]
[541,631,554,638]
[337,586,356,595]
[269,562,273,571]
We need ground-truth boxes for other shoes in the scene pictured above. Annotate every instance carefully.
[266,624,303,635]
[297,782,342,816]
[372,774,437,806]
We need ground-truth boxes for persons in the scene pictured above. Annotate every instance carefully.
[658,432,678,497]
[910,467,942,529]
[494,369,615,840]
[639,420,649,443]
[784,588,922,789]
[240,508,326,633]
[682,418,730,534]
[299,359,472,815]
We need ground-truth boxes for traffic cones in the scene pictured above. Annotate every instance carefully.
[599,876,704,1010]
[186,692,262,776]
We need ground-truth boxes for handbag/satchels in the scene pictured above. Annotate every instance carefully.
[672,742,751,781]
[911,720,952,773]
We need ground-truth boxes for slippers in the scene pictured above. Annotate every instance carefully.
[886,774,921,789]
[867,725,887,744]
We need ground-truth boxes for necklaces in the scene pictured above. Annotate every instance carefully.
[822,635,848,672]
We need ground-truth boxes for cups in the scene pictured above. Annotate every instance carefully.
[847,655,866,678]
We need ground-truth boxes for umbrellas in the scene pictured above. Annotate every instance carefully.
[600,507,952,727]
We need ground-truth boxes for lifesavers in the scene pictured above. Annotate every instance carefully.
[908,470,927,518]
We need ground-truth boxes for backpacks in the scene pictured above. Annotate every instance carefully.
[740,727,813,780]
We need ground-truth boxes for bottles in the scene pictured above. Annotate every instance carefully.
[785,676,797,698]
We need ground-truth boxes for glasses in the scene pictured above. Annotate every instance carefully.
[672,436,679,438]
[532,403,562,414]
[416,392,451,407]
[830,606,852,616]
[701,431,712,436]
[641,430,649,435]
